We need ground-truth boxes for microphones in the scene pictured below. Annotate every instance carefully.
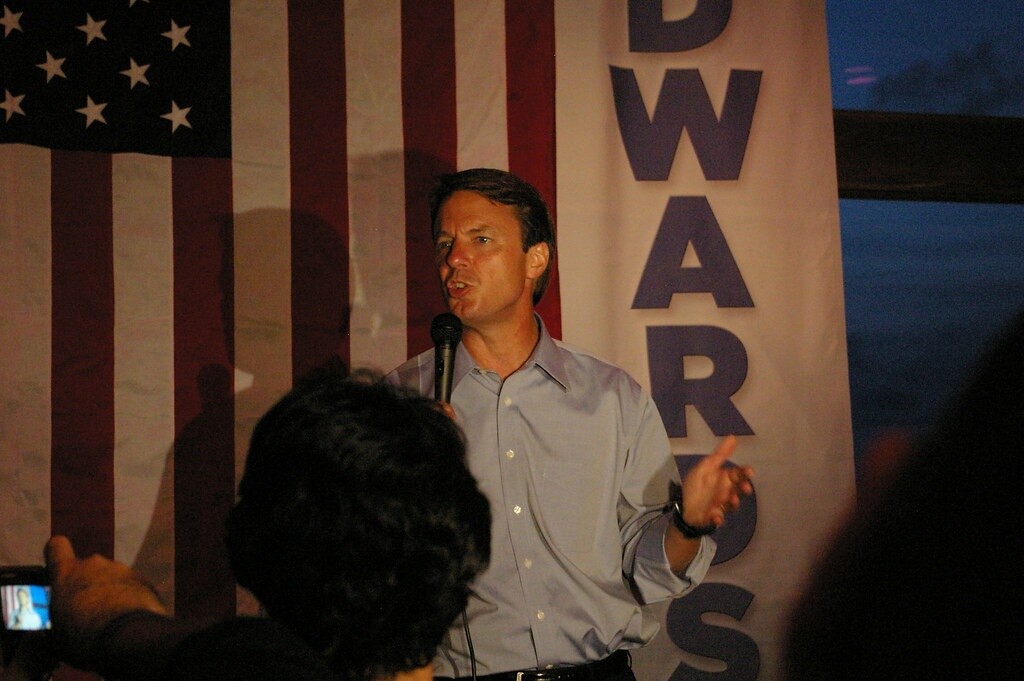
[430,311,464,404]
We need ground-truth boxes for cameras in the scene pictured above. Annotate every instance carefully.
[0,565,77,666]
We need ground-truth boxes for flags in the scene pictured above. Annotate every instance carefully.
[0,0,858,681]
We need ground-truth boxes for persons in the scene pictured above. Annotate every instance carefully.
[784,322,1024,680]
[43,534,338,680]
[10,590,41,630]
[227,372,491,680]
[381,167,754,681]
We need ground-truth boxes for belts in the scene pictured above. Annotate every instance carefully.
[461,649,627,681]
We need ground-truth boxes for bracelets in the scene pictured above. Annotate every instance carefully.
[667,482,714,538]
